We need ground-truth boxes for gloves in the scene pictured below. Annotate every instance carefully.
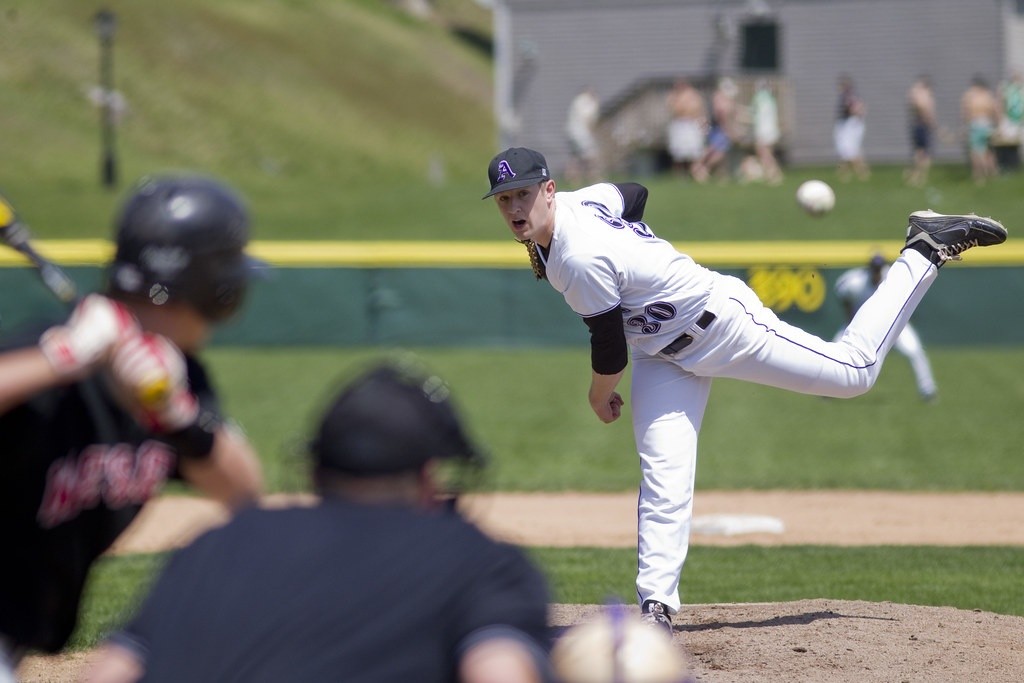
[39,296,139,376]
[103,336,200,433]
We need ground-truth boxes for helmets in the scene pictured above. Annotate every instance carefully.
[107,176,250,296]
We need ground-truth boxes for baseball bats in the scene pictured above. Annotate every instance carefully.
[0,198,170,406]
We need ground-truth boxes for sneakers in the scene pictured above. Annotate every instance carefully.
[903,209,1006,266]
[638,598,675,640]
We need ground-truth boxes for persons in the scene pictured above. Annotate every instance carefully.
[903,73,939,184]
[83,358,684,683]
[829,73,871,179]
[484,147,1010,634]
[828,253,937,399]
[0,169,269,683]
[960,70,1024,178]
[663,72,784,183]
[564,86,603,181]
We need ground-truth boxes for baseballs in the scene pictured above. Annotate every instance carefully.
[795,179,836,216]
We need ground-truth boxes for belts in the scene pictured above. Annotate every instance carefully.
[661,311,717,355]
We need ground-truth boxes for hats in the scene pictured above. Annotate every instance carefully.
[483,148,550,202]
[315,363,487,478]
